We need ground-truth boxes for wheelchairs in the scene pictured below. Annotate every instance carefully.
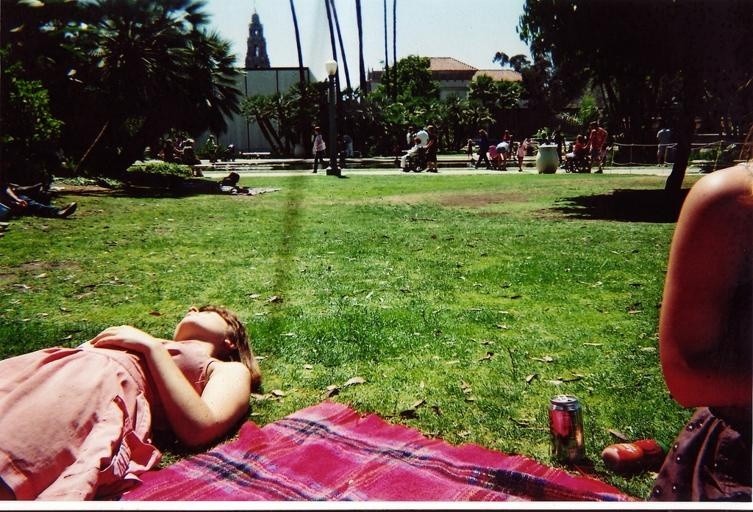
[404,147,431,172]
[560,145,592,173]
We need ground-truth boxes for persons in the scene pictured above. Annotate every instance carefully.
[656,124,671,163]
[648,162,753,501]
[159,128,203,177]
[467,128,528,171]
[206,135,237,165]
[406,124,438,172]
[537,122,611,173]
[313,127,353,173]
[0,303,262,501]
[0,173,78,220]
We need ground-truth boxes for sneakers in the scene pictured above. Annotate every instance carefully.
[594,170,602,173]
[57,202,77,217]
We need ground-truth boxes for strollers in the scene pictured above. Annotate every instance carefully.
[488,145,502,170]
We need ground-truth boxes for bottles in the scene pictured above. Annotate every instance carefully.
[602,440,664,471]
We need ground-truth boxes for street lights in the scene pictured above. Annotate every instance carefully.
[325,57,341,176]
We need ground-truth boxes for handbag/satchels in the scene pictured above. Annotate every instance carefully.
[317,142,324,151]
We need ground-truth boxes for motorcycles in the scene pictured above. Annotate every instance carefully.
[210,144,239,163]
